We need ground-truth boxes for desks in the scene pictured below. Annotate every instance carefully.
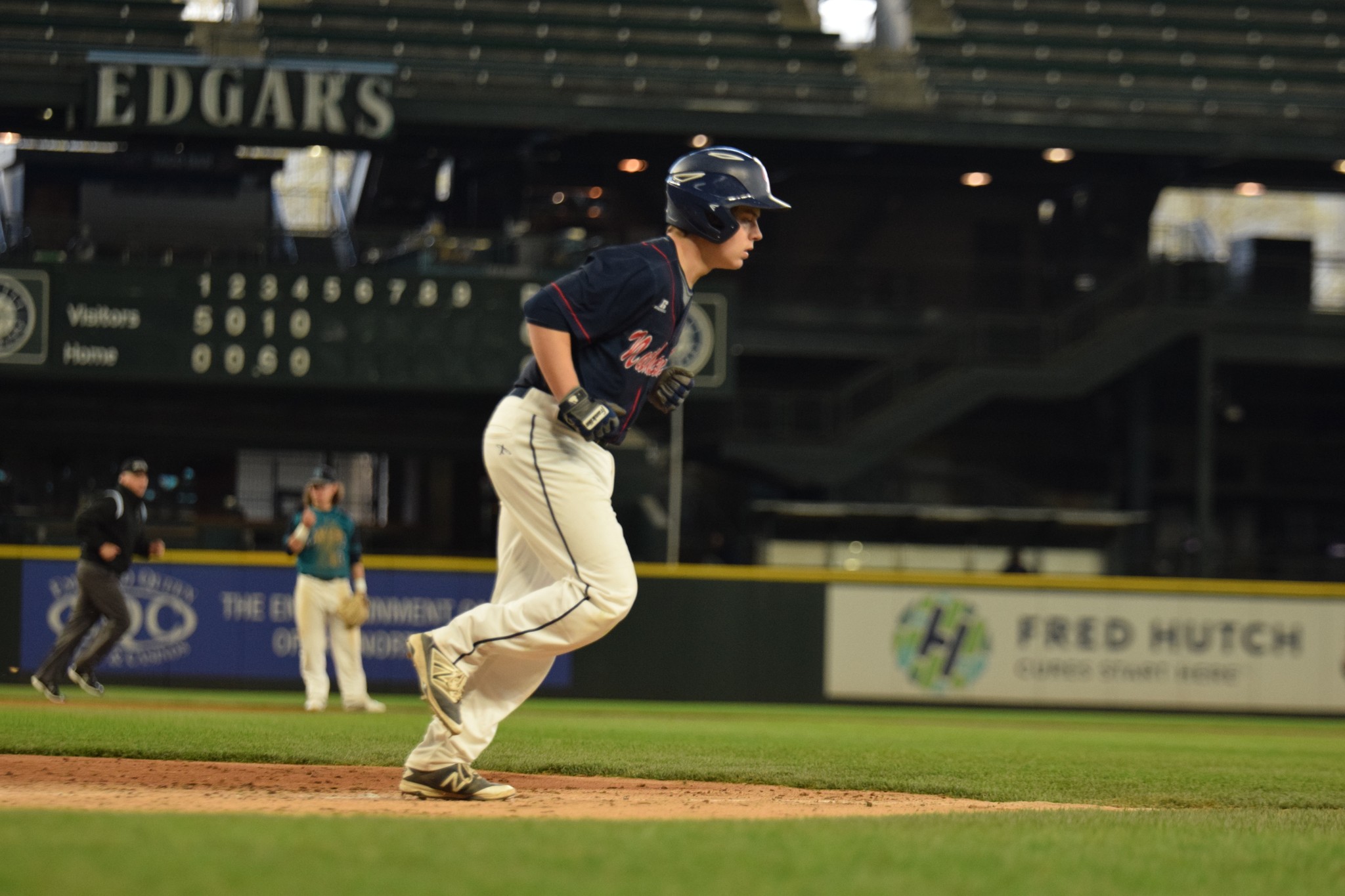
[750,496,1151,575]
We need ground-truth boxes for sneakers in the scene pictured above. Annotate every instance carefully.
[399,762,515,801]
[405,631,468,735]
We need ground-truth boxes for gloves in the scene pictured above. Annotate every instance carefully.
[647,366,694,414]
[558,387,627,442]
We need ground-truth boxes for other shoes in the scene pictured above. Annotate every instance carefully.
[31,675,64,704]
[343,697,386,714]
[304,700,327,713]
[68,664,104,696]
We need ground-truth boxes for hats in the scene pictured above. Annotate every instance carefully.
[310,464,338,486]
[122,459,148,476]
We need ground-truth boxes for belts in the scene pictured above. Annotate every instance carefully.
[509,386,609,449]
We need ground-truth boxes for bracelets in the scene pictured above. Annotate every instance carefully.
[353,577,368,593]
[294,521,310,544]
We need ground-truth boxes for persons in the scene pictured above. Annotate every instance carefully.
[396,146,792,801]
[282,464,386,716]
[30,455,167,705]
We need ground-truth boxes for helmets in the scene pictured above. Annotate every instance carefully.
[665,146,791,244]
